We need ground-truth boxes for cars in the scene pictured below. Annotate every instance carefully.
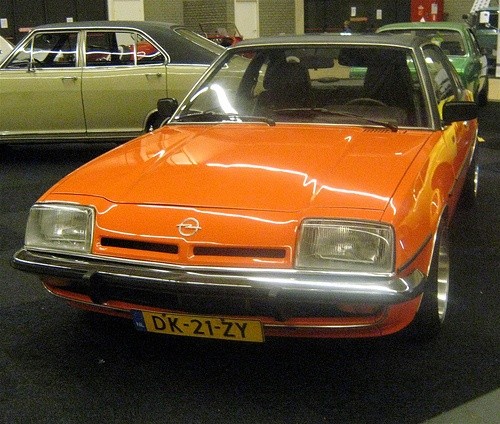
[12,35,480,343]
[350,21,493,100]
[0,20,260,144]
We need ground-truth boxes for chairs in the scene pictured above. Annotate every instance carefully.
[440,40,462,55]
[362,57,414,113]
[255,60,313,114]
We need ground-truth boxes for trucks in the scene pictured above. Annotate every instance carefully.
[462,8,498,53]
